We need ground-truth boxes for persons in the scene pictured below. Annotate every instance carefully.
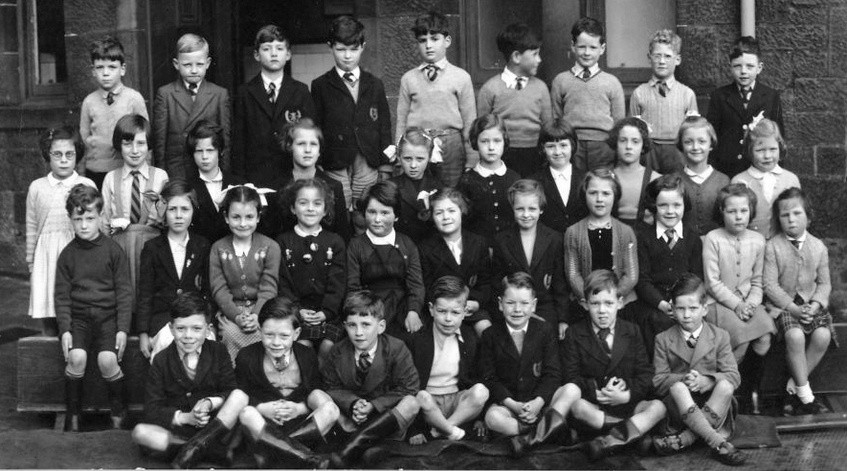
[53,184,131,434]
[706,36,784,180]
[130,291,249,469]
[26,125,99,338]
[136,12,730,364]
[558,269,667,464]
[652,271,749,465]
[78,34,150,194]
[765,187,840,418]
[100,114,170,296]
[233,295,339,470]
[317,289,421,470]
[408,275,490,444]
[730,110,800,240]
[475,272,582,462]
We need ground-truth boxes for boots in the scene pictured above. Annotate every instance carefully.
[588,420,641,461]
[105,377,129,424]
[65,375,82,423]
[160,408,568,470]
[598,414,654,456]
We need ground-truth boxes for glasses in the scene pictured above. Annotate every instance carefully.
[49,151,77,160]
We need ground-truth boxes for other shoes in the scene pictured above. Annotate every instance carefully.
[712,441,747,466]
[795,393,828,414]
[783,401,799,415]
[654,434,686,455]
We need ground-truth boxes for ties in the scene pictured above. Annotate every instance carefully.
[188,83,196,100]
[687,335,697,347]
[665,228,674,248]
[583,66,592,79]
[107,91,115,106]
[130,170,141,226]
[358,352,372,371]
[741,86,752,104]
[659,81,666,97]
[793,240,802,251]
[598,328,611,356]
[275,354,287,373]
[426,64,437,81]
[268,82,275,103]
[514,77,523,91]
[344,72,353,83]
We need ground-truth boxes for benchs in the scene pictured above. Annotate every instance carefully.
[13,327,149,432]
